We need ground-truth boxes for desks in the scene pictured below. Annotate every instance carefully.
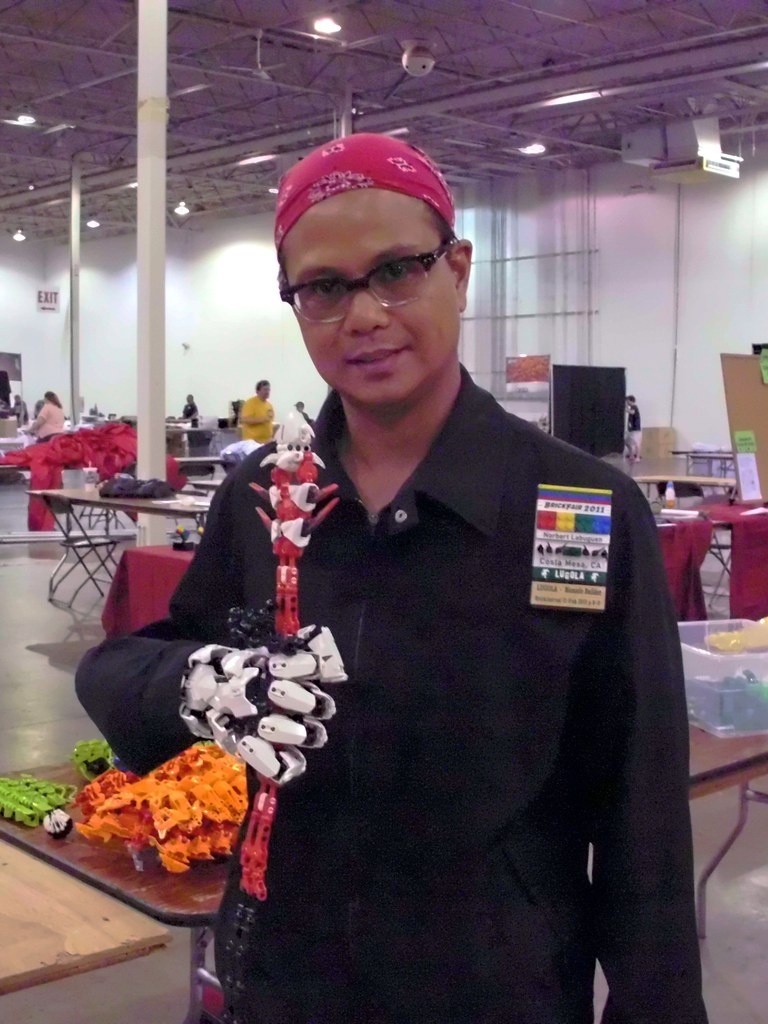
[0,414,280,602]
[100,545,198,643]
[0,725,768,1024]
[631,448,768,652]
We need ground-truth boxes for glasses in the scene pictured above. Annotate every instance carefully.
[280,246,447,322]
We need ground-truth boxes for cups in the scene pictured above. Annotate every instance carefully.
[83,467,97,492]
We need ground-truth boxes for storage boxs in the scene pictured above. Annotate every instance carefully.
[677,617,768,739]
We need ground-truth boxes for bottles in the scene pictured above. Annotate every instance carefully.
[664,481,675,510]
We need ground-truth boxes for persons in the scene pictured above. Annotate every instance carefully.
[73,135,717,1024]
[0,380,311,446]
[625,395,641,462]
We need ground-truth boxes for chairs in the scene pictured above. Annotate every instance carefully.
[41,492,118,608]
